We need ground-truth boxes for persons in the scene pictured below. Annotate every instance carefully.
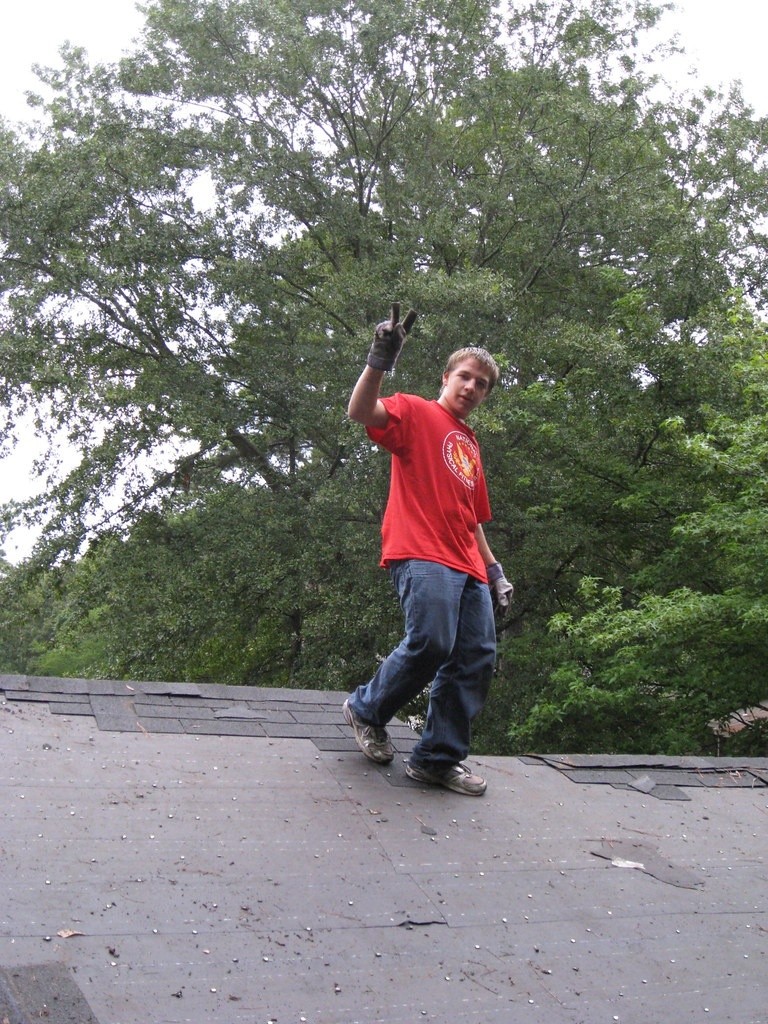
[342,303,513,795]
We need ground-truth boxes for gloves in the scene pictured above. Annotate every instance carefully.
[486,563,513,620]
[366,302,417,371]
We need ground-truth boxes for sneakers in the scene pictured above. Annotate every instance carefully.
[342,698,400,763]
[406,761,487,795]
